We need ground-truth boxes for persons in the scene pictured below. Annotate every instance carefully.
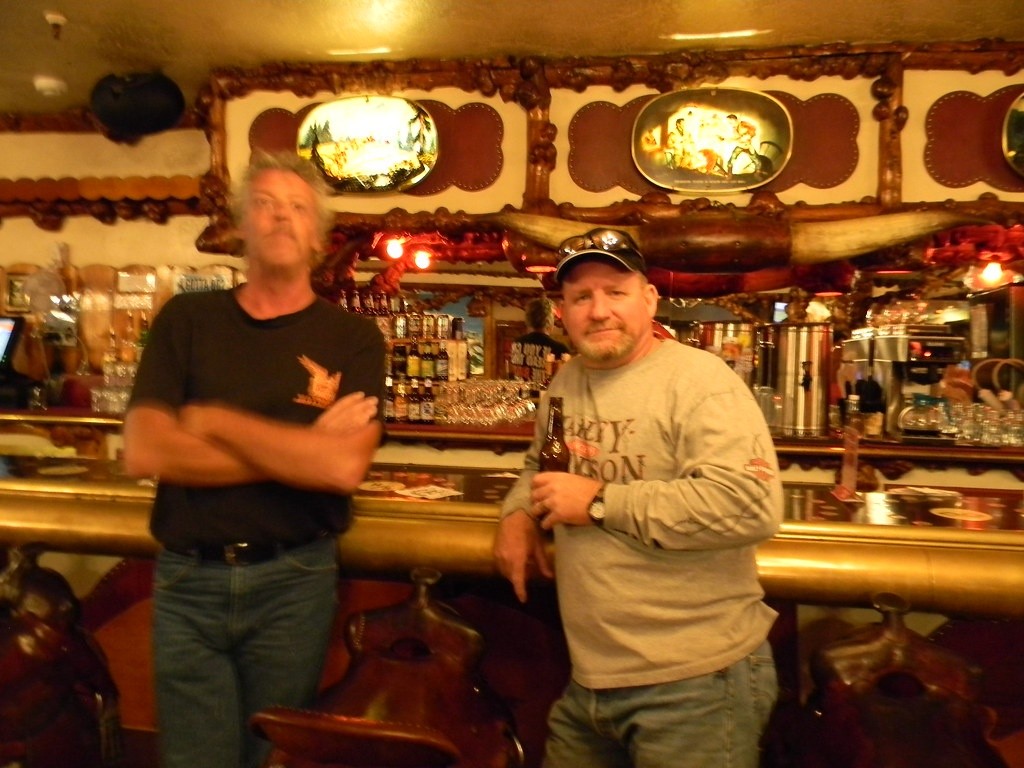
[123,152,387,768]
[495,226,782,768]
[508,297,570,383]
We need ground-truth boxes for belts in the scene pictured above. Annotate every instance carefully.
[162,526,328,564]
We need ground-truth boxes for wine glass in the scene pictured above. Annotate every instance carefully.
[931,401,1024,448]
[433,378,536,424]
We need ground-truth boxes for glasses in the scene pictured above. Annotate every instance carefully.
[556,227,645,264]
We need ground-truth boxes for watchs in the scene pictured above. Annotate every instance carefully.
[588,483,606,528]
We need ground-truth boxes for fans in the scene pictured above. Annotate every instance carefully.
[32,10,68,99]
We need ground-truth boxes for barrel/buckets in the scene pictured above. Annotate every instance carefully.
[755,320,831,441]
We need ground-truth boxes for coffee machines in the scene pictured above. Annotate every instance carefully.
[840,328,877,396]
[872,324,962,444]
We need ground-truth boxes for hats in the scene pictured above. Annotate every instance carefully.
[551,229,641,284]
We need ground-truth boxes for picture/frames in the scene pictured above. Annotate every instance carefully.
[296,95,439,194]
[631,86,792,193]
[1001,93,1023,172]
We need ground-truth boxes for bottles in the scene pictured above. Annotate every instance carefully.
[538,397,570,523]
[334,289,471,424]
[89,315,150,413]
